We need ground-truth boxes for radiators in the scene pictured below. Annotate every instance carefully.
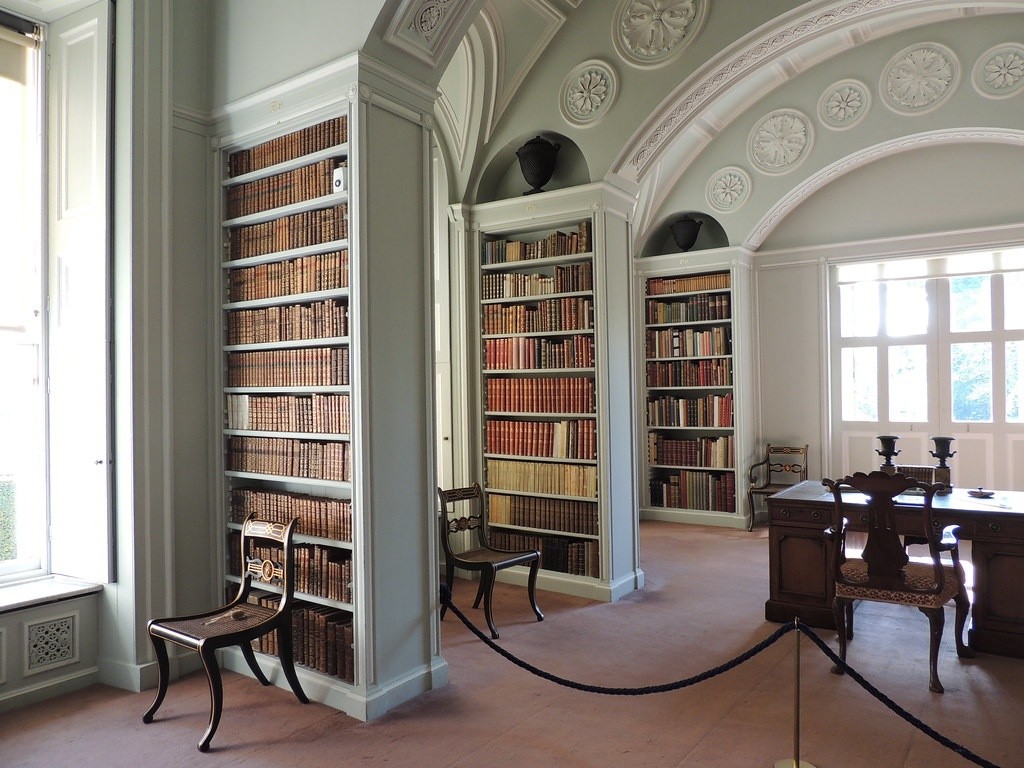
[897,465,934,485]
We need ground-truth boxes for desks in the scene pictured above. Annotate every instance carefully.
[764,479,1024,658]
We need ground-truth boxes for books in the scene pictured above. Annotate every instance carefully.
[226,116,355,682]
[645,273,736,513]
[482,222,598,577]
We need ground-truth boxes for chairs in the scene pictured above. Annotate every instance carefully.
[821,472,974,694]
[747,443,809,532]
[142,511,310,753]
[438,483,544,640]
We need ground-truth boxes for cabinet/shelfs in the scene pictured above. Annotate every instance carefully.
[640,263,757,529]
[471,202,645,604]
[208,86,450,722]
